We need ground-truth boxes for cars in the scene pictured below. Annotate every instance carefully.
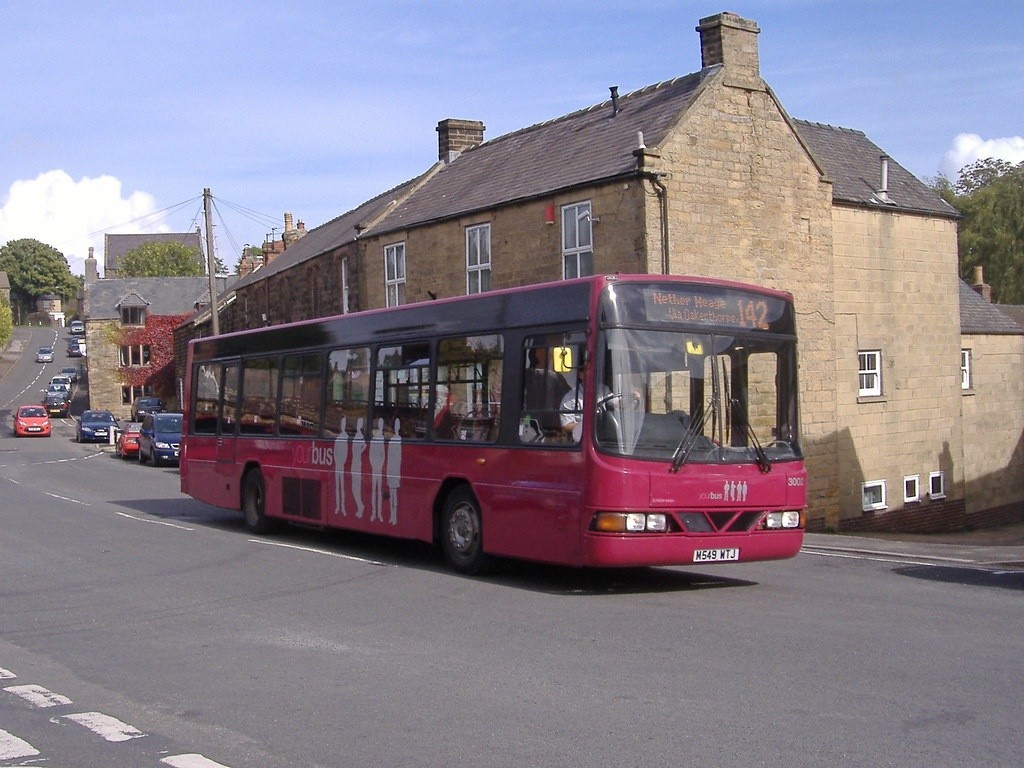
[130,395,168,421]
[13,404,52,437]
[67,320,85,358]
[116,422,144,459]
[36,347,54,362]
[43,367,77,417]
[74,409,121,443]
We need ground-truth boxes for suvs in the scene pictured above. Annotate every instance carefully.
[136,411,184,466]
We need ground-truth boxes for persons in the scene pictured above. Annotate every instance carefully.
[558,362,625,435]
[332,416,402,525]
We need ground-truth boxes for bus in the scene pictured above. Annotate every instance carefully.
[178,271,807,573]
